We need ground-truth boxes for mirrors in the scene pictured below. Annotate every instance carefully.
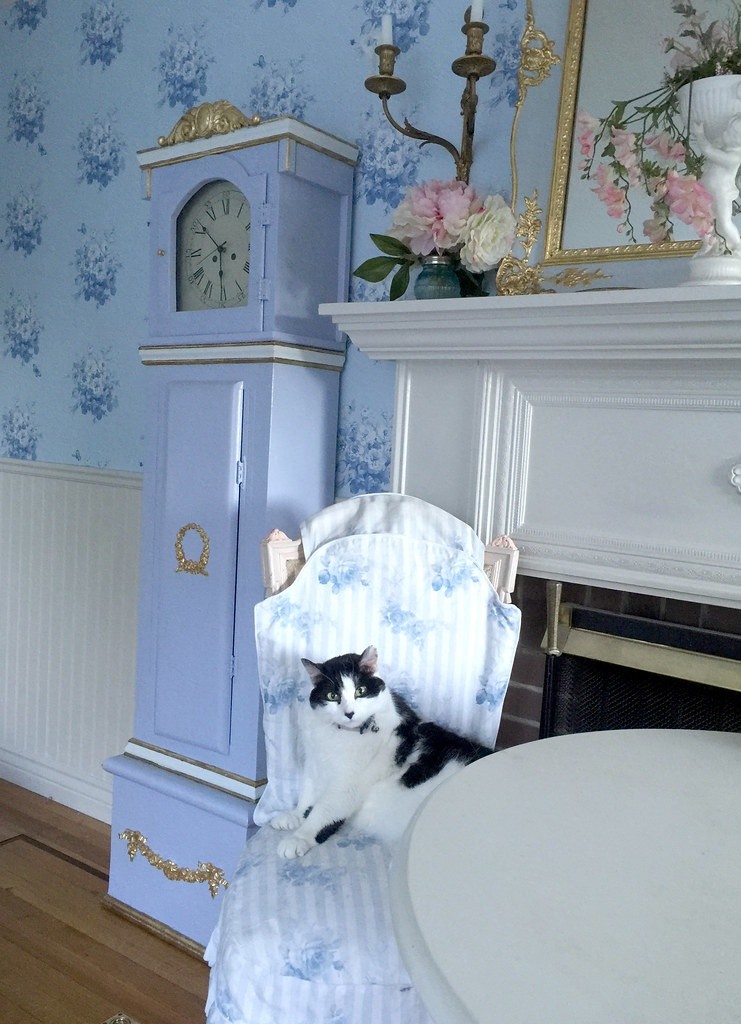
[494,0,741,296]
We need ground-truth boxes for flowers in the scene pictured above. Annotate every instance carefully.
[352,176,517,301]
[577,0,741,256]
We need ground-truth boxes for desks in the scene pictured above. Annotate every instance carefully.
[385,728,740,1024]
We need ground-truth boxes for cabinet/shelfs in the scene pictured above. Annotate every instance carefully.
[123,363,343,807]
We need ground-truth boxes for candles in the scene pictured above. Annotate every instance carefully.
[380,14,393,45]
[469,0,484,22]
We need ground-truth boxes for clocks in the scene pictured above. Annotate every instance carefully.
[135,101,362,352]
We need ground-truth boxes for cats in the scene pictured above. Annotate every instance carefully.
[273,644,499,866]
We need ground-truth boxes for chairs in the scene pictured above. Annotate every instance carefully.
[203,493,523,1023]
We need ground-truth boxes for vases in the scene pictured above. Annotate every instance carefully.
[414,256,462,299]
[676,74,741,140]
